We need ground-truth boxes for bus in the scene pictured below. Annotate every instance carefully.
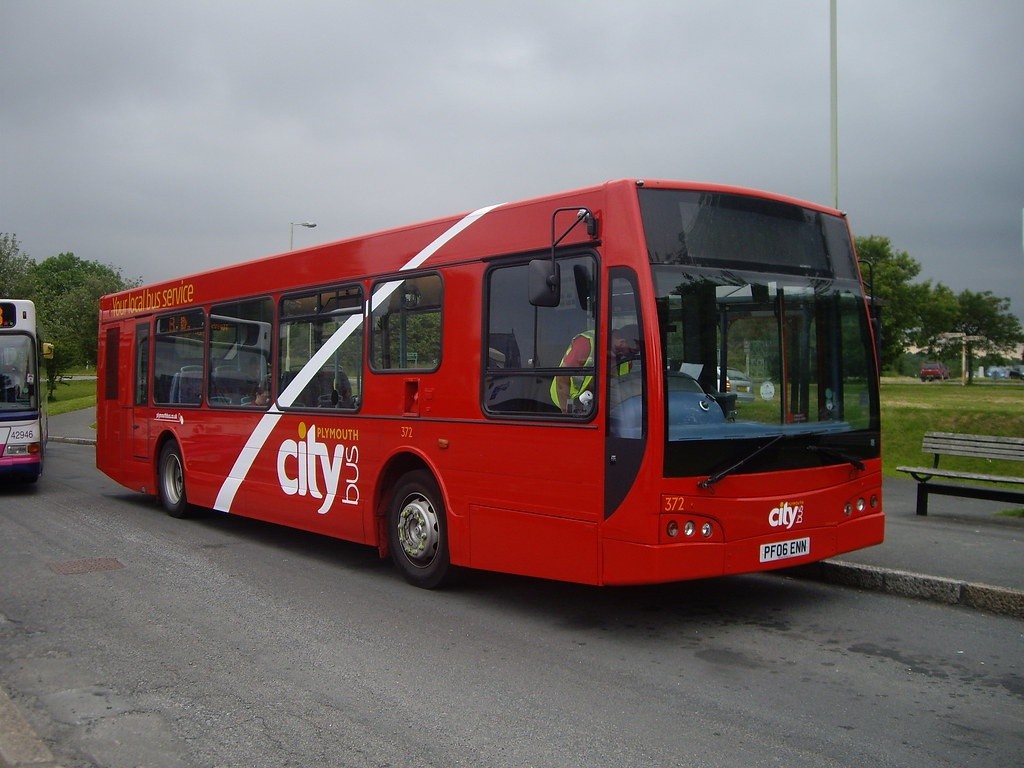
[93,178,888,591]
[1,299,55,483]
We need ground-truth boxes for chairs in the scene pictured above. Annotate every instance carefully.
[170,365,204,405]
[212,365,248,405]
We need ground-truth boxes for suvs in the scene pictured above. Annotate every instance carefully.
[715,365,756,404]
[921,360,951,382]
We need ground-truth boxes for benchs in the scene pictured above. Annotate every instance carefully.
[896,430,1024,516]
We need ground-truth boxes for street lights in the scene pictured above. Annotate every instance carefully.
[285,224,316,375]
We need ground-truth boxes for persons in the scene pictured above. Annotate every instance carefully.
[247,387,272,406]
[548,324,644,416]
